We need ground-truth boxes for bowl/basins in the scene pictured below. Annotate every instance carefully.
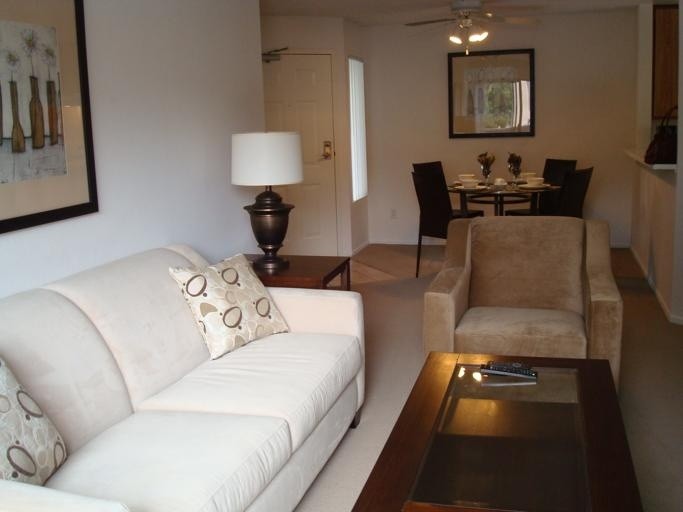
[457,172,545,188]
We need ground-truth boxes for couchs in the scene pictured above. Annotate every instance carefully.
[0,242,366,512]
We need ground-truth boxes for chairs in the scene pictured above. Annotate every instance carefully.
[421,216,623,399]
[410,159,594,277]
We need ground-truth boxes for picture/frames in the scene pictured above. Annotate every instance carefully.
[0,0,99,232]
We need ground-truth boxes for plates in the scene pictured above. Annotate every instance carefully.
[452,177,550,191]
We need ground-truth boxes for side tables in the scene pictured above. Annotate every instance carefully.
[242,253,350,292]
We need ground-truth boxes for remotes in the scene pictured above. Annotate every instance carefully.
[480,364,538,380]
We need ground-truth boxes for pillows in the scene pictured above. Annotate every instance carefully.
[172,254,290,362]
[0,356,67,487]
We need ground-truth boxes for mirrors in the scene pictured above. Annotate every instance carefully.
[447,49,536,138]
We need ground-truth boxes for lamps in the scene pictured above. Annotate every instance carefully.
[231,131,304,273]
[448,19,488,57]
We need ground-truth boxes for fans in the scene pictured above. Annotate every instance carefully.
[403,0,543,27]
